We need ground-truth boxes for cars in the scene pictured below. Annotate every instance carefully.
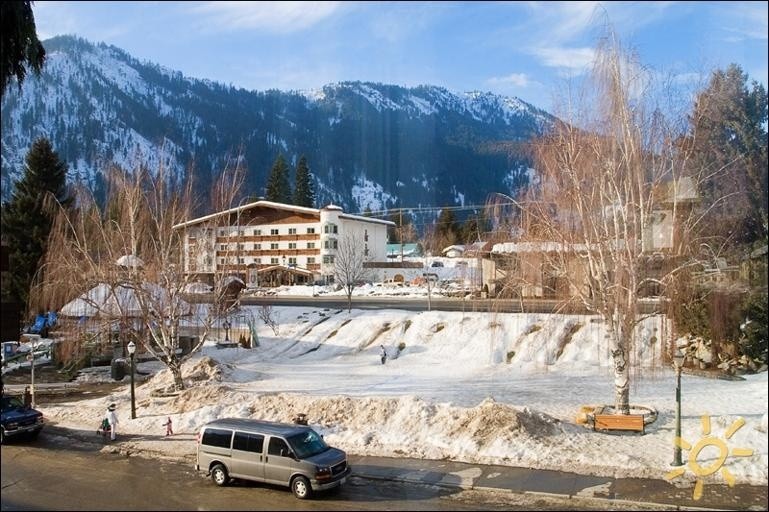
[0,394,46,444]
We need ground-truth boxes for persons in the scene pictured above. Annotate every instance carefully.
[23,385,33,409]
[106,404,119,441]
[161,416,173,435]
[378,344,387,364]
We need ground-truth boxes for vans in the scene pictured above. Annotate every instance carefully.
[196,418,353,500]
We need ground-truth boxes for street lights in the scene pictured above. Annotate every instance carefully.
[672,349,685,466]
[237,196,265,278]
[23,333,41,409]
[127,341,136,419]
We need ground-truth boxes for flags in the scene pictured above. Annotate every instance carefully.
[31,315,45,333]
